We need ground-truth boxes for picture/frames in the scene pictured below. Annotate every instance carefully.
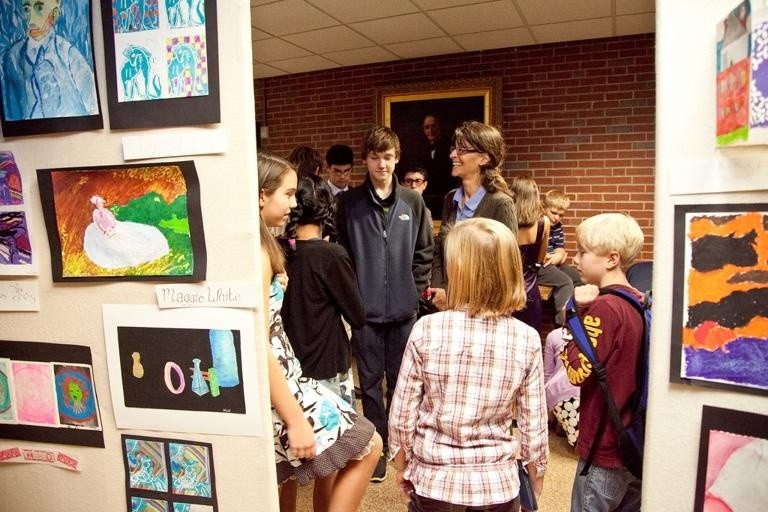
[371,75,502,236]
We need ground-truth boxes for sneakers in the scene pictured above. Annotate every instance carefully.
[370,451,387,481]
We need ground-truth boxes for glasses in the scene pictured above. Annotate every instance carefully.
[451,144,479,156]
[405,176,426,186]
[334,168,352,178]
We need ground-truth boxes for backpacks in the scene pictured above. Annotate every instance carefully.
[563,285,651,480]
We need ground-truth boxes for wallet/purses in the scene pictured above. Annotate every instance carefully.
[517,459,538,511]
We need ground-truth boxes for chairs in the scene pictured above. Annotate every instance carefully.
[626,261,652,292]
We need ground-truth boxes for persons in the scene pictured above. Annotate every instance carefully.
[416,115,453,161]
[258,121,650,512]
